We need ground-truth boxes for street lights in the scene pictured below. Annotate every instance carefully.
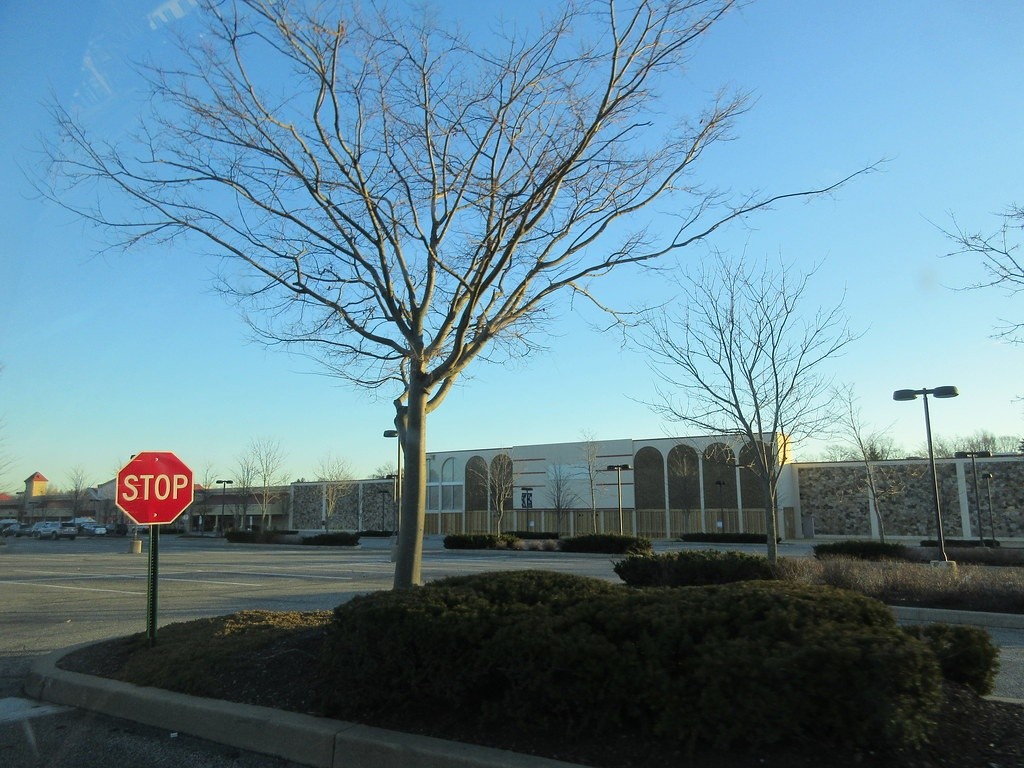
[382,428,403,545]
[982,472,998,547]
[954,448,993,547]
[215,479,234,525]
[27,501,39,523]
[716,479,729,534]
[89,499,100,520]
[889,382,960,560]
[608,462,633,535]
[522,487,534,531]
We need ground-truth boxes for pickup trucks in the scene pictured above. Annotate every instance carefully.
[35,522,79,541]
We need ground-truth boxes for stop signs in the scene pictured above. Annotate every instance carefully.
[114,452,193,525]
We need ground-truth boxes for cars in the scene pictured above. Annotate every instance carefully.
[2,523,34,537]
[131,525,150,534]
[0,518,18,531]
[81,523,107,536]
